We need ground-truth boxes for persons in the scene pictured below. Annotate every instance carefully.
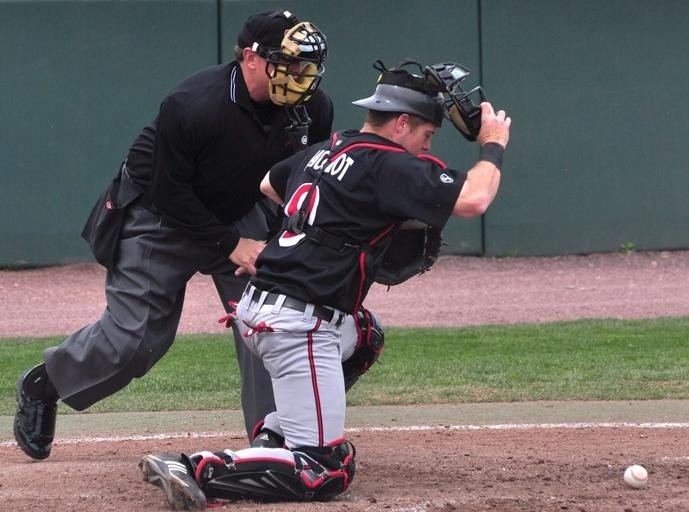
[137,57,513,511]
[9,5,334,463]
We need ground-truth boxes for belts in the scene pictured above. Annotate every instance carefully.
[245,283,343,327]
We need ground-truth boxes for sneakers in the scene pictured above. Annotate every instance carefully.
[140,452,209,511]
[13,363,57,460]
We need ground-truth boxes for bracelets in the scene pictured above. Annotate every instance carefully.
[479,142,504,172]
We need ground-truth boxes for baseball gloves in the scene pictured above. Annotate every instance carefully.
[375,216,448,292]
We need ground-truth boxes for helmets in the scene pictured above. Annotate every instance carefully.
[351,64,448,127]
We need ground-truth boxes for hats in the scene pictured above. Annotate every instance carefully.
[238,9,325,66]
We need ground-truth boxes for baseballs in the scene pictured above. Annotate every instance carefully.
[624,464,647,488]
